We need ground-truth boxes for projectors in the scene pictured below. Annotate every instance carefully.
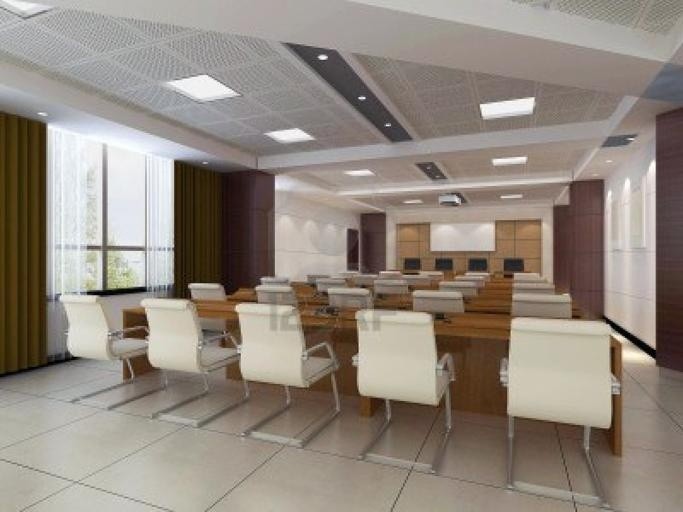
[439,195,462,207]
